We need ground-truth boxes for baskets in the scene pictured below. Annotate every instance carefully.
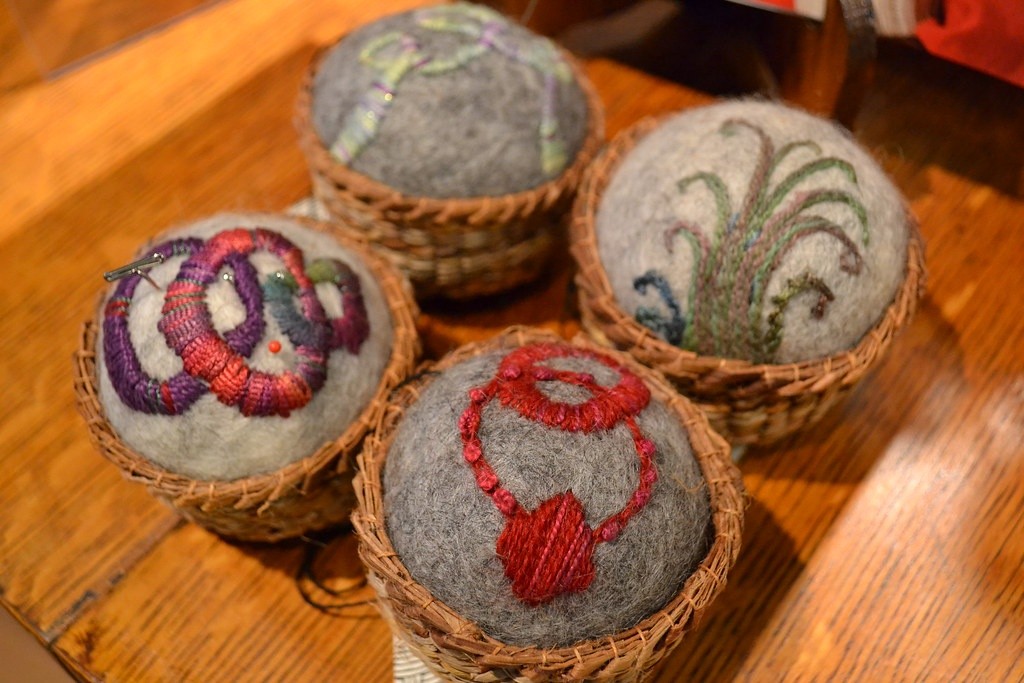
[353,324,746,683]
[292,32,605,299]
[72,207,425,543]
[571,105,920,446]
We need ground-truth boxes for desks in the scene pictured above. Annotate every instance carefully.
[0,0,1023,683]
